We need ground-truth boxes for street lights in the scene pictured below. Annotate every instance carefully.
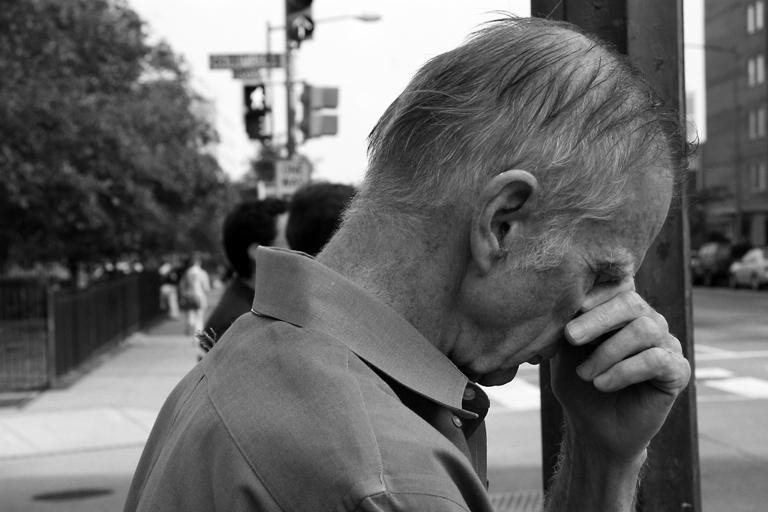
[266,14,383,105]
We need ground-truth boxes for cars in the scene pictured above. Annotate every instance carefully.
[691,241,768,290]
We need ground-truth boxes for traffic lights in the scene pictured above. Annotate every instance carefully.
[303,85,339,138]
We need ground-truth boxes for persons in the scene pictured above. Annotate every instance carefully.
[201,199,291,352]
[180,251,209,336]
[287,180,359,264]
[156,258,179,320]
[125,17,693,512]
